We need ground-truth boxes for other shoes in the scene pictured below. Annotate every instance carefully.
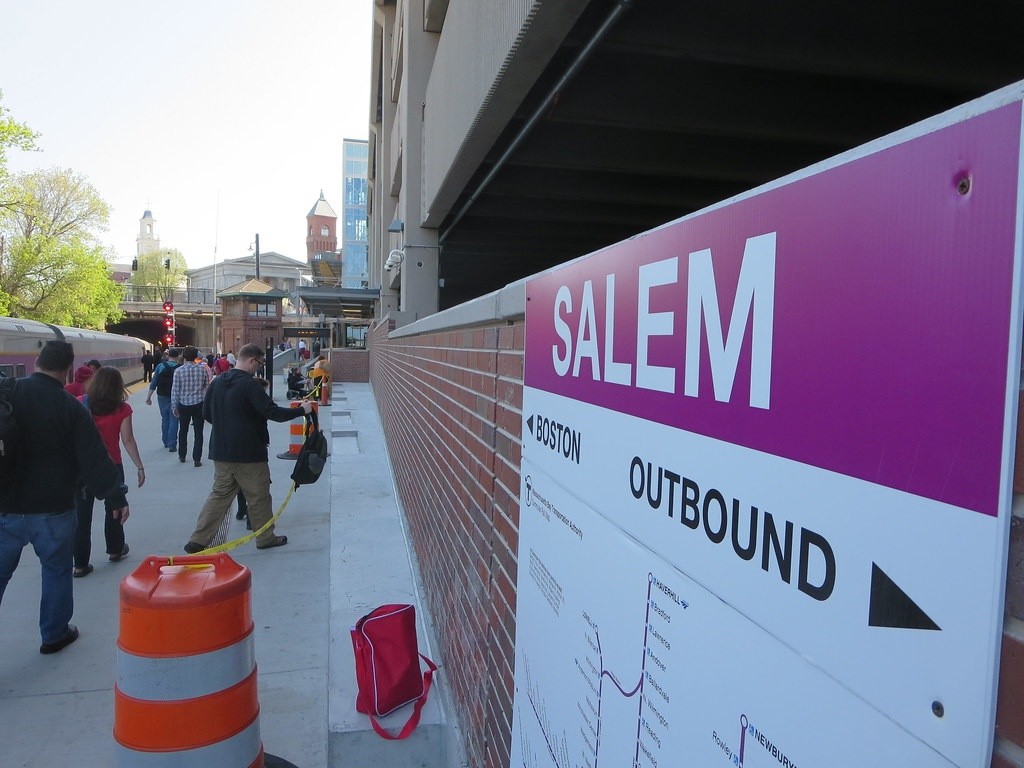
[179,455,185,463]
[183,542,205,554]
[246,518,252,530]
[255,534,287,549]
[73,564,94,577]
[235,505,247,519]
[169,448,177,452]
[194,460,202,467]
[108,543,129,561]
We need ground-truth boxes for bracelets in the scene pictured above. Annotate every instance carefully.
[138,468,144,470]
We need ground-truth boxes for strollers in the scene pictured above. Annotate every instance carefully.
[286,361,316,400]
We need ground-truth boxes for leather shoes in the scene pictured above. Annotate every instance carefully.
[40,624,79,654]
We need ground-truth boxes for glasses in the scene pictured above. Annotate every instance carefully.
[250,356,264,367]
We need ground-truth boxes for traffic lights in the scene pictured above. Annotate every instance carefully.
[163,298,173,312]
[132,259,137,271]
[165,259,170,270]
[162,316,174,329]
[163,333,175,344]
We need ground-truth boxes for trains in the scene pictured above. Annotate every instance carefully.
[0,314,154,386]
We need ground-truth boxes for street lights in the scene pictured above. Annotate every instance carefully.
[294,266,303,314]
[247,233,260,279]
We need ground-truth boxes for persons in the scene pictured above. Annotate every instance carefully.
[72,365,145,577]
[235,377,269,530]
[170,347,210,467]
[87,360,102,373]
[1,340,130,655]
[63,366,94,399]
[183,342,313,554]
[145,347,185,452]
[140,337,329,401]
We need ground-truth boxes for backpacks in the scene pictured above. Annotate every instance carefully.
[157,362,181,397]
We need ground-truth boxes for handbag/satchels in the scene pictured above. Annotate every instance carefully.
[290,401,327,492]
[0,376,24,506]
[348,603,438,740]
